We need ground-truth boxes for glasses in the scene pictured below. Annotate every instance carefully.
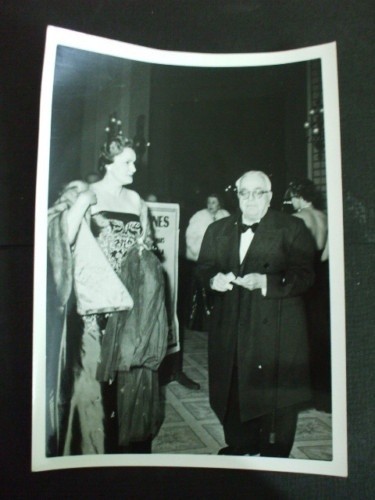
[239,188,270,200]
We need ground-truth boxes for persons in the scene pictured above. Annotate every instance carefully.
[282,179,331,416]
[44,134,170,454]
[195,170,319,460]
[184,194,232,322]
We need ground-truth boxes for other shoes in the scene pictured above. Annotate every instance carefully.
[218,445,259,456]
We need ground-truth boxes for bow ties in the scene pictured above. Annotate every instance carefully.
[240,222,259,234]
